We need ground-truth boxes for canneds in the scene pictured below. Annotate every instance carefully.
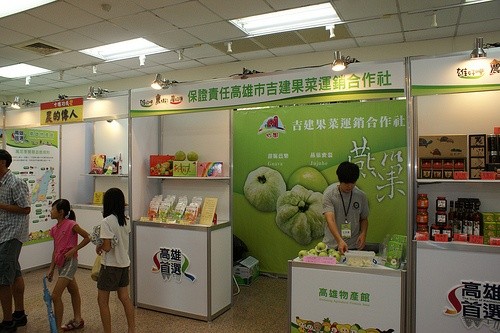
[417,193,452,242]
[487,135,500,163]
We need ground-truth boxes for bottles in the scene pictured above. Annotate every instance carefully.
[112,157,116,174]
[448,200,481,240]
[488,135,500,163]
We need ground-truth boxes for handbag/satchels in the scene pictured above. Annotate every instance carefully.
[90,254,101,281]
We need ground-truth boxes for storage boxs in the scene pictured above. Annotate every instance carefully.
[481,212,500,245]
[150,155,224,178]
[418,133,500,182]
[234,255,259,287]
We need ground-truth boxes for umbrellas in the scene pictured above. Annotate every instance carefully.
[43,276,57,333]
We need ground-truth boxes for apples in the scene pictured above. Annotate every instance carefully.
[298,242,341,262]
[175,151,186,161]
[187,152,198,161]
[287,166,328,194]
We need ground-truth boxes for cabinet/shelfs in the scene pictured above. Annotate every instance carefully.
[412,95,500,246]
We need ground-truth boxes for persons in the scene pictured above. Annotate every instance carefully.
[96,188,135,333]
[322,161,369,256]
[0,149,31,333]
[46,199,92,333]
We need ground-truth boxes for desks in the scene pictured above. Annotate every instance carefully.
[287,257,407,333]
[71,203,130,270]
[132,219,233,322]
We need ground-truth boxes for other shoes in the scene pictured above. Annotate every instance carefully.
[11,313,28,327]
[0,319,18,333]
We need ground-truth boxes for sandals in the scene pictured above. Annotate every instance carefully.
[62,318,84,331]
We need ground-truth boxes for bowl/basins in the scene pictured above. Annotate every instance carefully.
[344,250,375,267]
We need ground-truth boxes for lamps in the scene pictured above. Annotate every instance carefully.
[139,55,145,66]
[224,41,232,53]
[25,77,30,85]
[11,96,21,109]
[86,86,97,100]
[58,70,64,80]
[470,37,486,59]
[176,49,184,60]
[92,65,97,73]
[151,74,165,89]
[332,51,346,71]
[431,14,437,28]
[326,26,336,39]
